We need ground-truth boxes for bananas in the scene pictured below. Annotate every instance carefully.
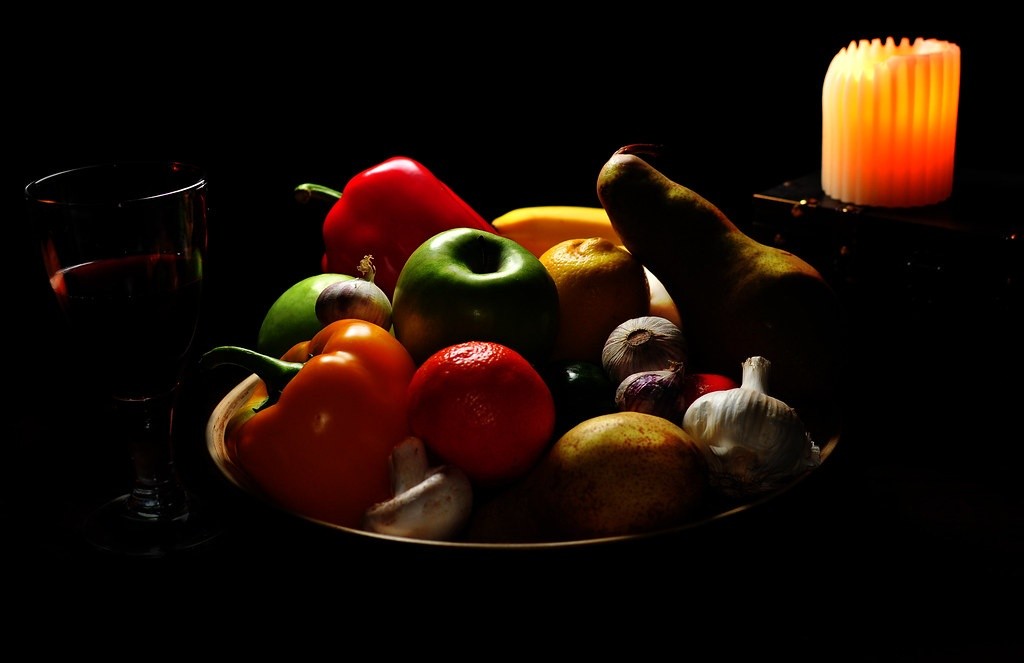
[488,204,624,259]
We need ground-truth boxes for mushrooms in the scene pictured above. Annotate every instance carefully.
[363,436,472,540]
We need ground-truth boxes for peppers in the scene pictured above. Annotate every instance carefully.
[295,157,499,303]
[199,319,418,528]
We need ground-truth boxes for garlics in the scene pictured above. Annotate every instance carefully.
[600,314,813,494]
[314,256,393,329]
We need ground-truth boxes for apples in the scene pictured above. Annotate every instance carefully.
[390,227,561,384]
[251,273,394,400]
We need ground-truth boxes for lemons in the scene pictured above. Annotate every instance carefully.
[535,238,651,375]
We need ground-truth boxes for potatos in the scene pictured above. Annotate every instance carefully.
[597,152,838,394]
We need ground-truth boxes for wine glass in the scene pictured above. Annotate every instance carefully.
[23,161,226,556]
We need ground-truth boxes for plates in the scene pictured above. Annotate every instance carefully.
[205,372,841,551]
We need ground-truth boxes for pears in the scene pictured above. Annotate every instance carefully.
[533,412,716,536]
